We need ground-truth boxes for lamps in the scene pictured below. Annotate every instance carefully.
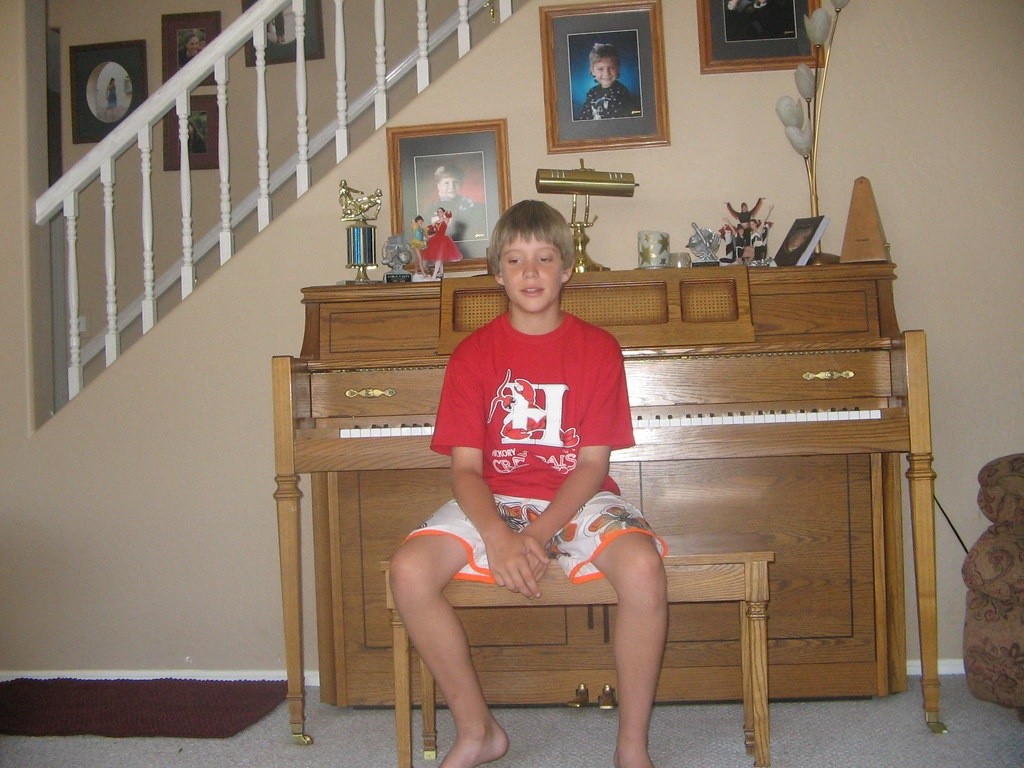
[534,158,640,272]
[776,0,850,266]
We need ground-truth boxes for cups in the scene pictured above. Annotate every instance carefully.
[637,230,670,269]
[669,252,692,269]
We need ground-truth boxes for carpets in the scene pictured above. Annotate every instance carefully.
[0,677,307,739]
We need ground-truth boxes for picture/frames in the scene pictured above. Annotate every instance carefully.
[161,11,221,86]
[162,94,219,171]
[68,39,148,144]
[539,0,671,155]
[385,118,512,273]
[696,0,825,75]
[241,0,325,68]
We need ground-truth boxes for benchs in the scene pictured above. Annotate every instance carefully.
[369,532,776,768]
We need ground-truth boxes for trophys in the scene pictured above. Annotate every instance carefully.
[382,233,411,283]
[339,180,382,285]
[686,222,719,267]
[536,157,638,273]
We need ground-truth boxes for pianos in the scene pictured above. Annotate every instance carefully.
[270,263,949,746]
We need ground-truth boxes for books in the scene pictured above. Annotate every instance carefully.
[773,216,829,266]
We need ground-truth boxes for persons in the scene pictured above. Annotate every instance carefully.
[103,78,117,119]
[578,43,630,120]
[411,215,434,278]
[424,206,462,279]
[434,164,465,206]
[719,197,774,264]
[178,34,199,66]
[275,11,285,42]
[392,200,670,768]
[724,0,777,39]
[189,122,206,153]
